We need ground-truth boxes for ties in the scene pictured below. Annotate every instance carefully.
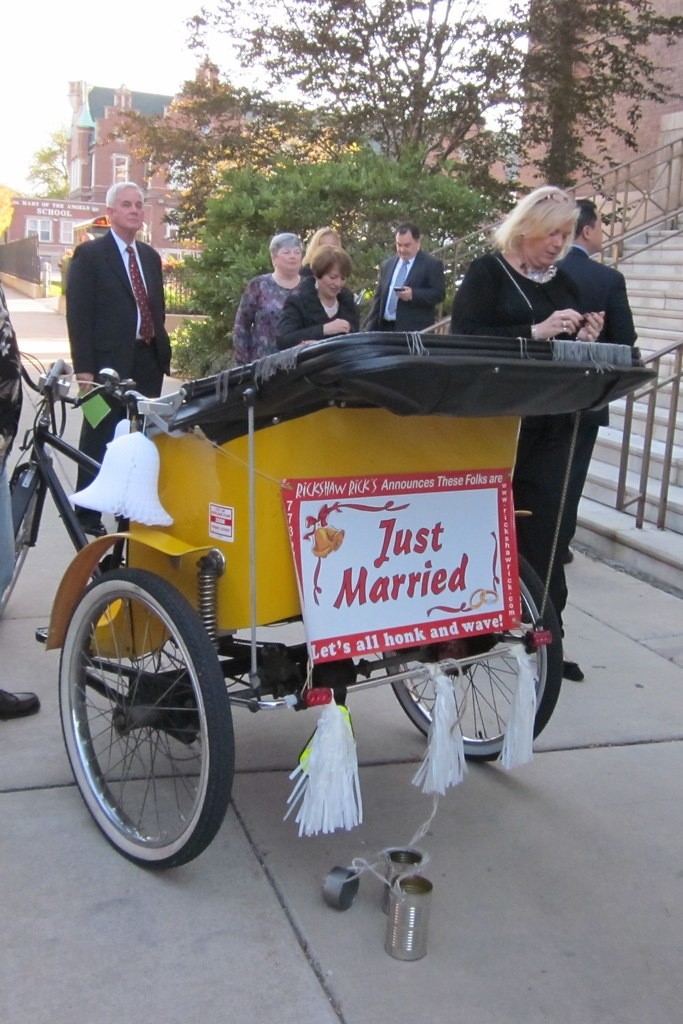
[387,257,412,314]
[126,245,156,347]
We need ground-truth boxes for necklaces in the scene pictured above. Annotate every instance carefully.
[512,252,557,283]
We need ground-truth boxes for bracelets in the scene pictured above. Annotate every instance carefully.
[532,324,538,342]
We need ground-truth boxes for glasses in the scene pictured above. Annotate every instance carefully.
[532,193,575,207]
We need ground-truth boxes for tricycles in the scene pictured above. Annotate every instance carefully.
[0,328,660,873]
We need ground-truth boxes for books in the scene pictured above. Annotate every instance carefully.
[76,385,111,429]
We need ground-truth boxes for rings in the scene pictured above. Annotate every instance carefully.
[563,321,567,326]
[560,313,565,318]
[563,327,567,332]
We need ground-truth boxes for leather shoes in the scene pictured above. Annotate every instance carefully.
[0,687,40,720]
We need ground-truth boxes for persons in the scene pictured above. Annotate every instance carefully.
[275,245,360,352]
[548,200,639,637]
[232,233,308,367]
[365,224,446,334]
[450,186,605,342]
[64,182,172,529]
[302,226,343,279]
[0,268,41,720]
